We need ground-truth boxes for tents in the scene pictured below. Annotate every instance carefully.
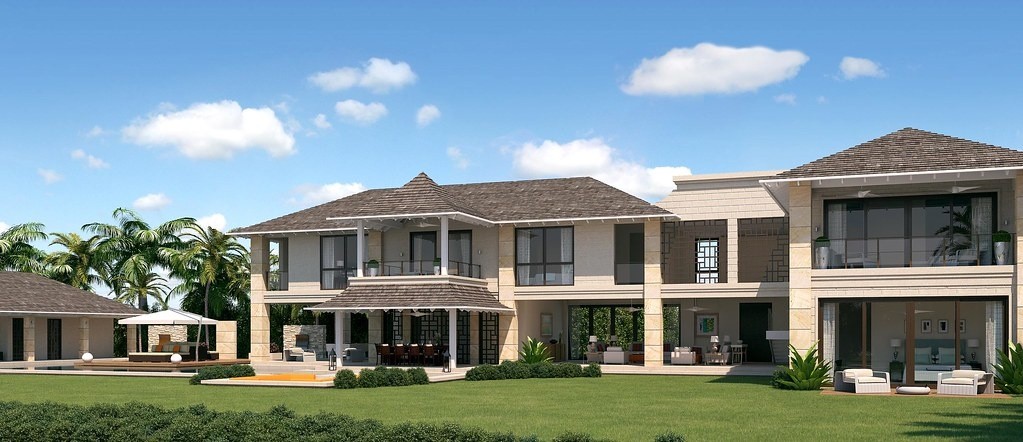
[117,307,216,362]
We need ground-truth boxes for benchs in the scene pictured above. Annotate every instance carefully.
[325,344,350,360]
[207,350,219,360]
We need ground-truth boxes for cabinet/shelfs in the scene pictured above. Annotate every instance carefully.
[545,343,566,363]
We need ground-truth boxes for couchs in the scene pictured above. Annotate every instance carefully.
[704,345,730,366]
[937,370,994,394]
[284,347,316,362]
[904,362,971,381]
[671,346,696,365]
[584,345,603,364]
[835,368,890,394]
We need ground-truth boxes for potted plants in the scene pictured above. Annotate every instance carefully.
[433,258,441,275]
[814,236,830,269]
[992,230,1011,265]
[368,260,379,276]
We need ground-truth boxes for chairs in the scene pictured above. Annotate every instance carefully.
[394,343,405,366]
[374,342,390,366]
[629,342,644,363]
[956,232,979,266]
[841,251,865,269]
[408,343,420,366]
[423,343,434,366]
[664,342,673,361]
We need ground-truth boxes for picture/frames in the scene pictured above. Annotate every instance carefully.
[938,319,949,334]
[694,312,719,338]
[960,319,966,334]
[921,319,933,334]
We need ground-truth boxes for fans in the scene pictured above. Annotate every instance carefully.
[623,299,642,314]
[407,219,440,228]
[681,298,710,312]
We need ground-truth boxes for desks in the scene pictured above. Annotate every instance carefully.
[705,353,723,366]
[604,352,624,365]
[731,344,748,365]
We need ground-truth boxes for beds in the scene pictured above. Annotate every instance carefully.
[128,344,190,362]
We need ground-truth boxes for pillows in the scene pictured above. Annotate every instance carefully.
[939,348,954,365]
[173,345,180,352]
[914,347,932,365]
[633,344,642,352]
[663,344,670,352]
[155,345,163,352]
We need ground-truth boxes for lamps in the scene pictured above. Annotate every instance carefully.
[858,186,865,197]
[337,261,344,289]
[611,336,617,347]
[589,336,597,353]
[710,336,719,353]
[967,338,980,363]
[952,181,960,193]
[890,338,901,361]
[724,335,731,345]
